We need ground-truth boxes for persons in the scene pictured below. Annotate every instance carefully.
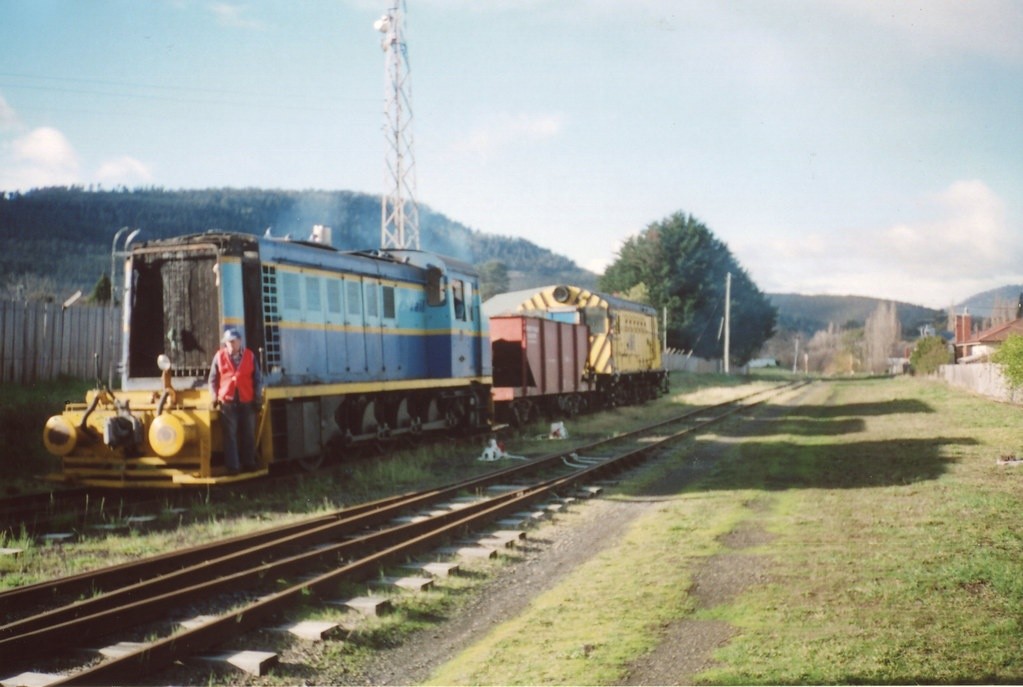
[209,329,262,475]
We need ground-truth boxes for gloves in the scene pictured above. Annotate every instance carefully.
[211,401,222,411]
[257,404,262,412]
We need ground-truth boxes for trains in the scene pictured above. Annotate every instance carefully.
[33,225,671,488]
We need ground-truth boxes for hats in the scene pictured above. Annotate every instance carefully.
[221,329,241,343]
